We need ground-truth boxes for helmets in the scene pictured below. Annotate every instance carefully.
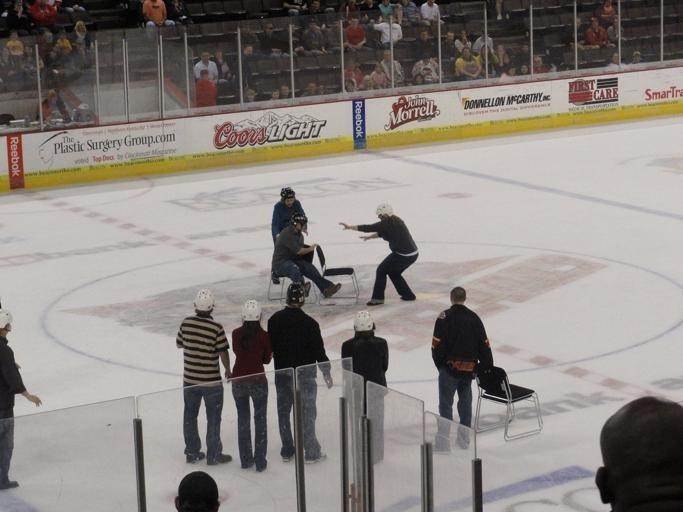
[0,308,15,329]
[240,298,262,325]
[193,288,216,312]
[352,309,376,334]
[285,281,305,305]
[289,211,308,229]
[280,185,298,202]
[375,202,396,218]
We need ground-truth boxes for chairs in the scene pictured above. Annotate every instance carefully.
[1,1,162,88]
[503,0,683,73]
[266,245,317,307]
[474,367,543,441]
[309,243,360,307]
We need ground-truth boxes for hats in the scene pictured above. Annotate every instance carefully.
[176,470,220,506]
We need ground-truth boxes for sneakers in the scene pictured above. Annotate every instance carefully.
[420,437,453,455]
[255,460,267,472]
[1,479,21,490]
[185,451,206,463]
[240,457,255,469]
[352,450,386,466]
[281,449,297,463]
[300,450,328,465]
[206,452,233,466]
[323,283,342,297]
[456,435,472,449]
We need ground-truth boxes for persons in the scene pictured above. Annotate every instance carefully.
[271,212,342,298]
[595,396,683,512]
[176,289,234,464]
[116,0,195,40]
[190,22,218,57]
[1,0,96,125]
[271,186,308,284]
[268,282,334,464]
[430,287,493,453]
[338,203,420,306]
[1,309,43,489]
[195,1,642,108]
[341,311,389,464]
[174,470,220,512]
[230,300,274,473]
